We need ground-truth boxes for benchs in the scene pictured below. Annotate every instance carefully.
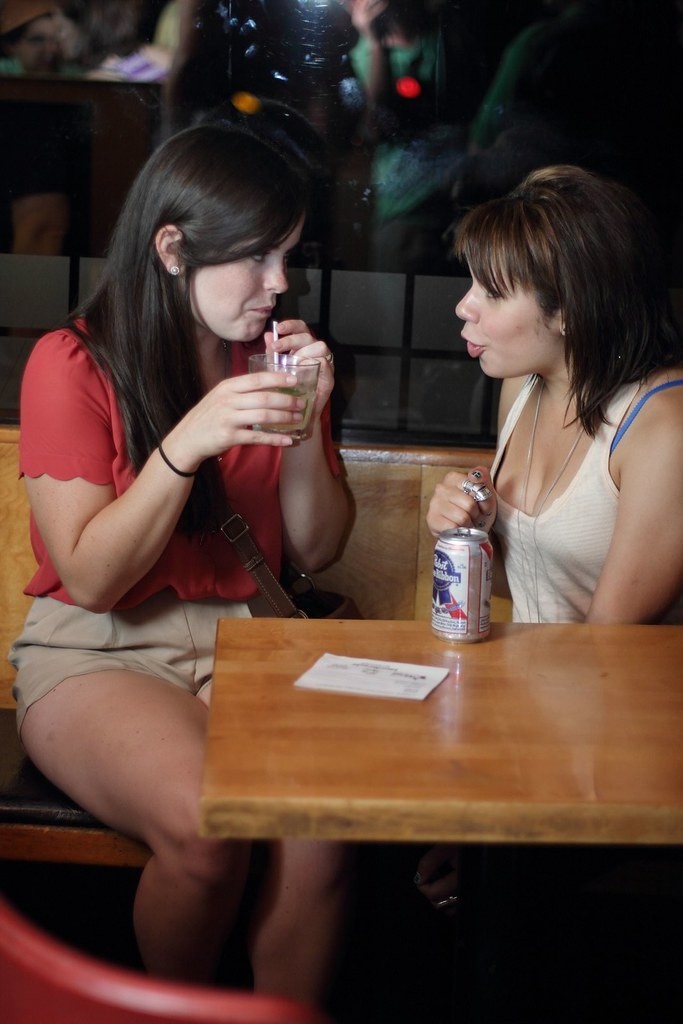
[1,427,514,870]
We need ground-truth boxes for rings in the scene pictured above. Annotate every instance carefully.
[412,872,424,885]
[462,479,494,501]
[326,353,334,364]
[437,896,462,909]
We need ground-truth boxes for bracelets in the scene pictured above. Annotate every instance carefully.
[158,441,197,478]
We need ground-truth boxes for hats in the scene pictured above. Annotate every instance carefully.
[0,0,61,36]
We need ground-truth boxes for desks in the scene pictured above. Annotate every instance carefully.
[199,618,682,844]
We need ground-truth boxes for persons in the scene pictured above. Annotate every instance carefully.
[413,163,683,1024]
[8,128,349,1012]
[0,0,683,279]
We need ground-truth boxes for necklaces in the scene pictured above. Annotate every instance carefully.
[517,382,583,623]
[223,340,228,378]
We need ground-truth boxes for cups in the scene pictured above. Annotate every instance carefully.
[248,353,320,441]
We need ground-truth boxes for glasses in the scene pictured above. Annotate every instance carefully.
[20,35,51,47]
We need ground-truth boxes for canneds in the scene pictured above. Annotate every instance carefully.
[430,526,495,644]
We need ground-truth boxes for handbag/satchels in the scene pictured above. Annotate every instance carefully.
[290,572,363,621]
